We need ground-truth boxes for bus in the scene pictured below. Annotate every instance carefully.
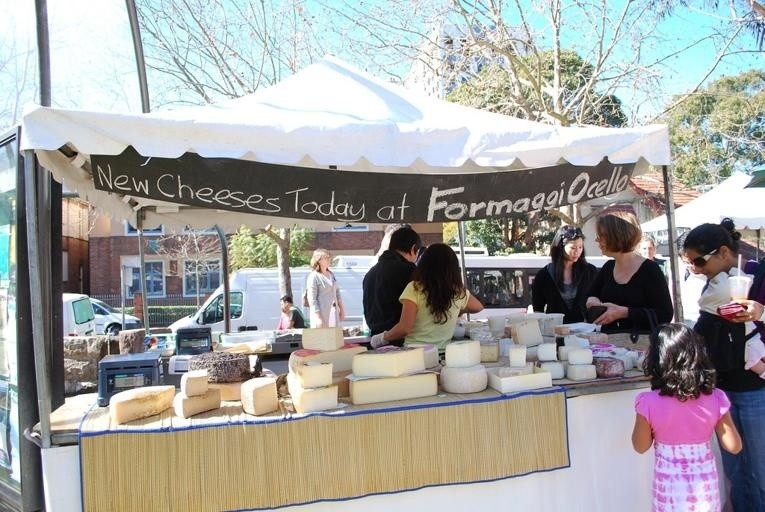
[454,253,613,323]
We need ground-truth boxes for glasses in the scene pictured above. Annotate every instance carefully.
[561,228,583,240]
[693,250,716,268]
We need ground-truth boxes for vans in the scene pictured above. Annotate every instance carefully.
[92,297,142,334]
[62,292,97,335]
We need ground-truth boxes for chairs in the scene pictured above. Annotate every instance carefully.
[231,293,243,320]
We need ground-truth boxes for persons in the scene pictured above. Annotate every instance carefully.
[306,248,345,328]
[362,226,421,346]
[280,295,305,329]
[580,204,674,334]
[276,303,290,330]
[531,225,598,323]
[639,230,765,347]
[371,242,483,362]
[632,321,741,511]
[685,219,765,511]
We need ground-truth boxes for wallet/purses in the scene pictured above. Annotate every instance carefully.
[717,302,745,318]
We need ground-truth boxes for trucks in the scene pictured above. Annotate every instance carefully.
[167,266,368,335]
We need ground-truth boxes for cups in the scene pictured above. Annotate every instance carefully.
[728,276,751,301]
